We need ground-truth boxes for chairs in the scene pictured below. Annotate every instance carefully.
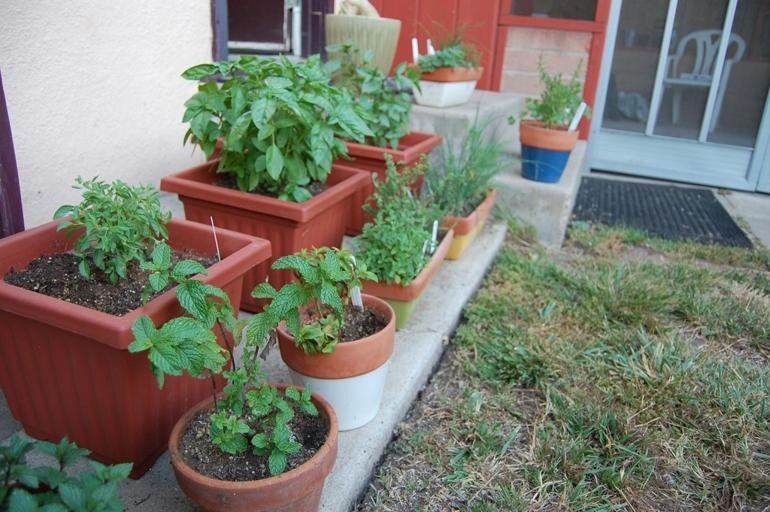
[649,28,748,143]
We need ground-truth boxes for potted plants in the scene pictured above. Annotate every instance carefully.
[5,178,273,481]
[262,243,398,429]
[159,50,367,315]
[167,349,340,512]
[515,64,584,182]
[428,109,503,262]
[348,150,455,331]
[341,35,442,238]
[411,35,484,109]
